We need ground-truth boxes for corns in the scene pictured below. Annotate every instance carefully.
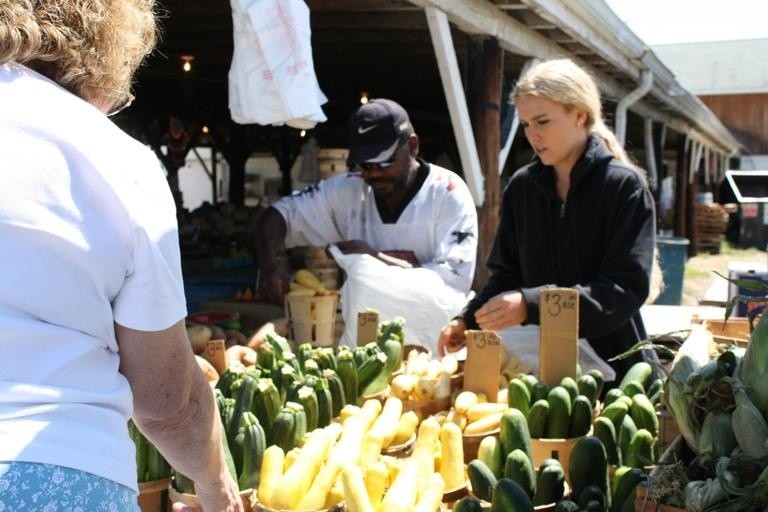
[664,302,768,512]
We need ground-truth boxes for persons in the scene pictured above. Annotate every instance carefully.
[250,97,481,299]
[1,0,244,510]
[435,57,667,404]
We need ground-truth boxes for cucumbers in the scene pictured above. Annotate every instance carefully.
[127,269,660,512]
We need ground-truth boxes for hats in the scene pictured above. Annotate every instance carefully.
[345,98,414,167]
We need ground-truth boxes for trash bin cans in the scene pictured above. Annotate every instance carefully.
[652,236,690,304]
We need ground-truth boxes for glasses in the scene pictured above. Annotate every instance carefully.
[354,157,394,172]
[105,90,135,117]
